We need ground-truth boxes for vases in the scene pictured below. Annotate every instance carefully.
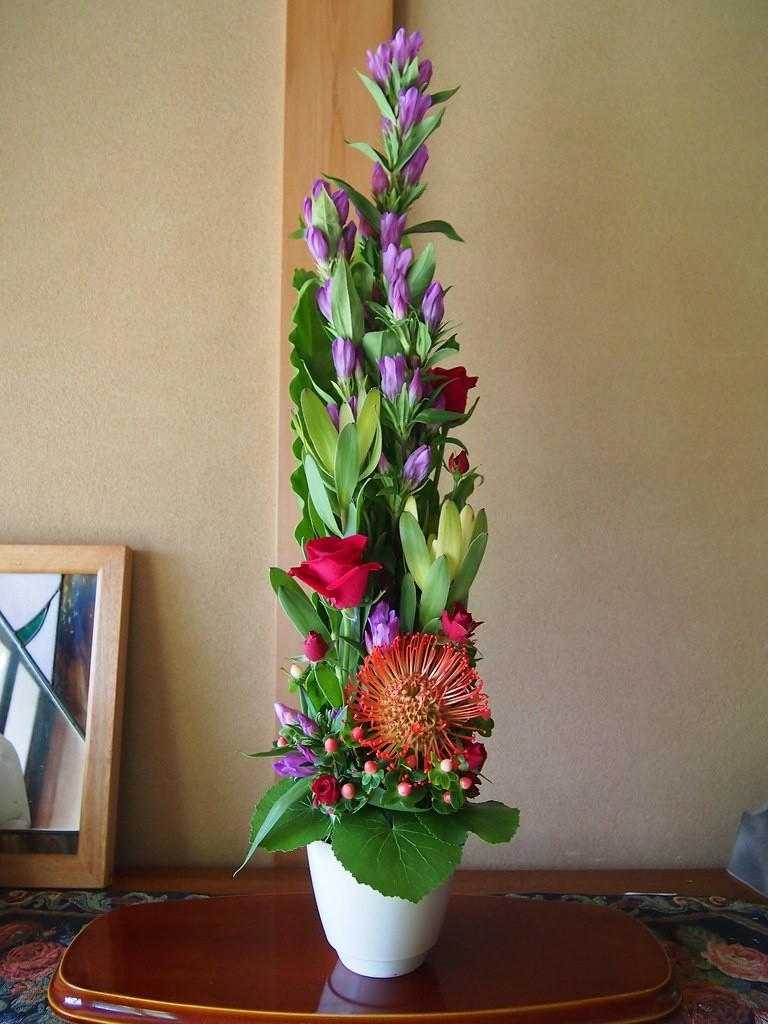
[304,837,454,980]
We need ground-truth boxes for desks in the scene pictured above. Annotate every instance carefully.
[47,894,685,1024]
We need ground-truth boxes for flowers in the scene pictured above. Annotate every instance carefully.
[233,22,521,906]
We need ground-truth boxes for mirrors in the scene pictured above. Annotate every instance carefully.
[1,542,135,892]
